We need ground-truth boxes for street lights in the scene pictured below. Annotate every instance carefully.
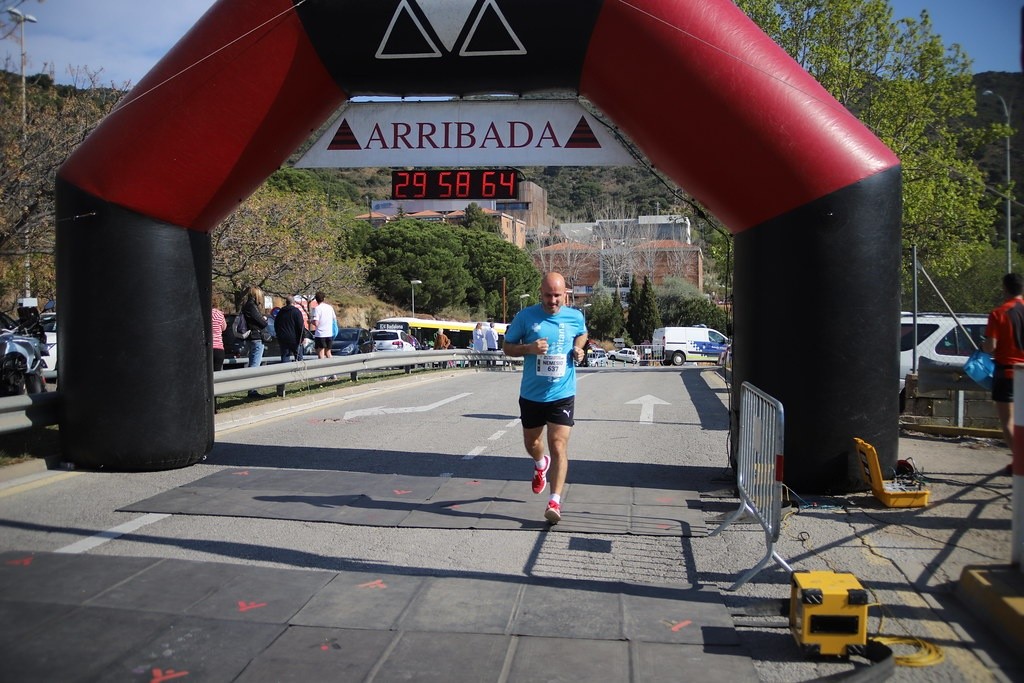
[584,304,592,318]
[411,280,422,317]
[521,294,530,310]
[6,4,39,295]
[983,89,1012,274]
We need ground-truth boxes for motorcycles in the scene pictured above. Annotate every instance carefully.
[0,300,56,392]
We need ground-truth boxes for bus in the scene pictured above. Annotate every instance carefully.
[375,317,511,351]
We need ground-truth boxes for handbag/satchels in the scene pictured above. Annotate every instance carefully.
[962,343,995,390]
[259,326,273,343]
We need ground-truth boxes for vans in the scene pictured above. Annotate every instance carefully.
[651,324,731,367]
[899,310,991,413]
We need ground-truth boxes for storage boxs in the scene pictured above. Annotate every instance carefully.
[788,570,868,657]
[854,437,930,508]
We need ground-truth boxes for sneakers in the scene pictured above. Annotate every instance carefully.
[531,455,551,494]
[544,500,562,525]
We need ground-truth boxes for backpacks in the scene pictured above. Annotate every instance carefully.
[232,306,253,342]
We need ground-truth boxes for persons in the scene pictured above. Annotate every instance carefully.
[434,327,455,369]
[270,297,283,319]
[211,294,227,371]
[242,285,269,400]
[503,272,589,524]
[310,290,339,381]
[485,322,499,369]
[422,338,430,350]
[274,294,304,363]
[980,273,1024,450]
[469,323,485,368]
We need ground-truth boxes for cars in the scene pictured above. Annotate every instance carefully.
[586,342,609,367]
[220,313,284,357]
[304,327,318,355]
[607,347,640,364]
[13,311,57,381]
[369,330,415,352]
[330,328,374,357]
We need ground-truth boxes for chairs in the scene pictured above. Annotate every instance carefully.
[352,334,357,340]
